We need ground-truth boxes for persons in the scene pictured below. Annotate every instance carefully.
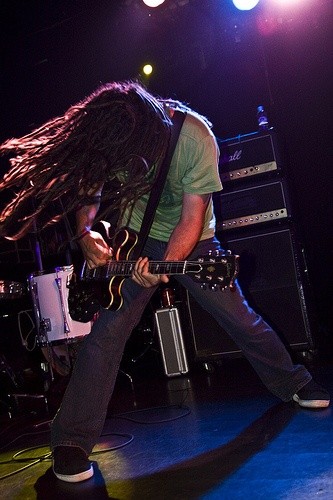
[0,81,330,482]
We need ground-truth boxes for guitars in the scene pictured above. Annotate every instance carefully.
[66,219,240,324]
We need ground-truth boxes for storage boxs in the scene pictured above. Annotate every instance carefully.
[155,308,188,377]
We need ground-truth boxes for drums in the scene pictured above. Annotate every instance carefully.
[29,264,94,349]
[0,279,31,354]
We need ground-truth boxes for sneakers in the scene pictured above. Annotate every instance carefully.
[52,447,94,482]
[293,380,331,407]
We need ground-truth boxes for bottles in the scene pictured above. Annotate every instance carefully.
[256,106,270,129]
